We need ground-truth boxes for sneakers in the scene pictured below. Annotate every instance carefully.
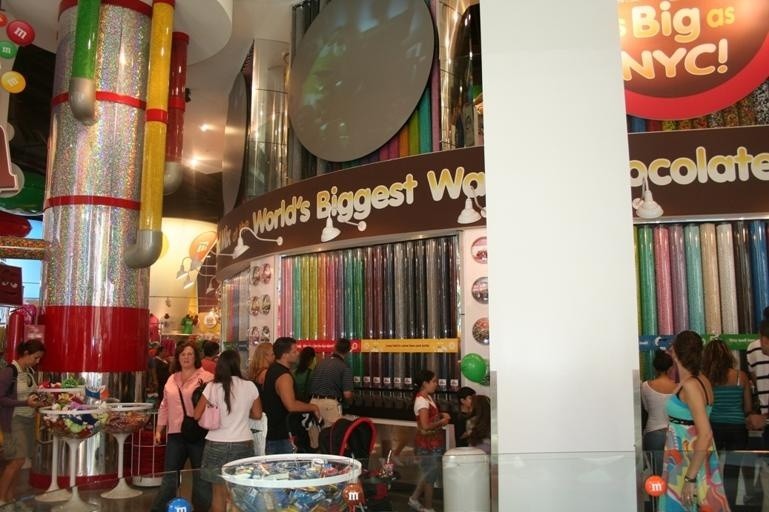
[407,496,434,512]
[0,497,34,512]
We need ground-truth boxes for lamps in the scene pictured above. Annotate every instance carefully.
[189,242,231,271]
[319,206,366,241]
[457,183,486,226]
[175,255,216,279]
[205,275,215,296]
[183,270,215,289]
[231,226,283,260]
[631,173,665,219]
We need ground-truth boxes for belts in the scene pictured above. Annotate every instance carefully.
[313,393,339,400]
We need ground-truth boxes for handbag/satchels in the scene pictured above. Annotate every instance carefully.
[181,415,209,442]
[417,413,442,435]
[198,382,221,430]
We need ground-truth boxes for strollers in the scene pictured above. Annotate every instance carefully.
[312,416,392,512]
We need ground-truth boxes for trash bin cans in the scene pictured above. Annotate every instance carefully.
[442,446,490,512]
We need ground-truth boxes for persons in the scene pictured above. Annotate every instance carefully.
[642,305,769,511]
[409,369,491,512]
[0,340,47,507]
[39,373,60,388]
[0,352,8,369]
